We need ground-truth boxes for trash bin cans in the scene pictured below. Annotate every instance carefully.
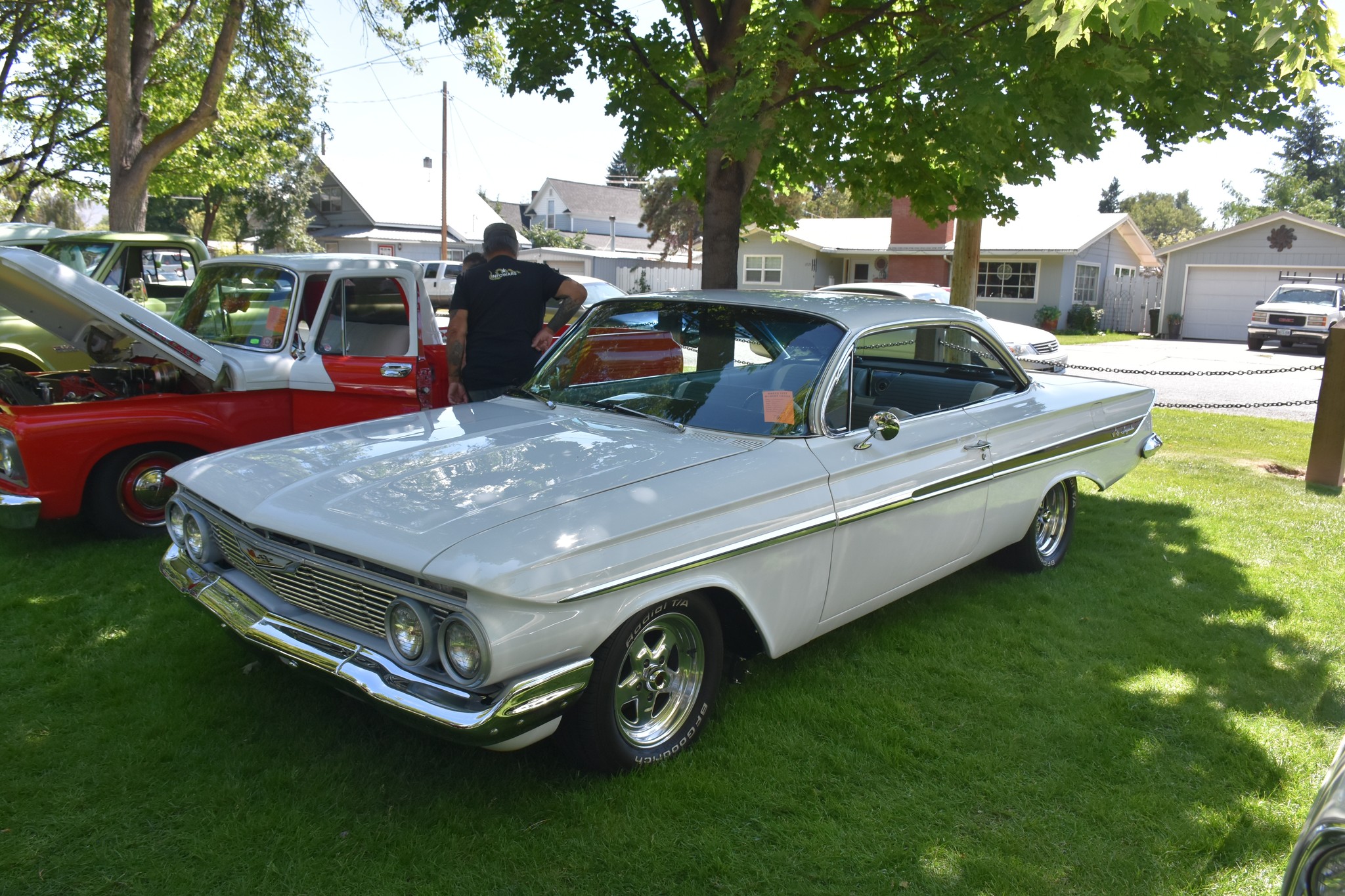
[1149,308,1162,334]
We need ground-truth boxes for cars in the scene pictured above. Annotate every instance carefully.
[541,274,701,348]
[751,281,1068,375]
[86,252,196,291]
[0,222,86,275]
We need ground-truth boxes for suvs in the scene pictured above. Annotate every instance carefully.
[417,261,464,314]
[1247,284,1345,356]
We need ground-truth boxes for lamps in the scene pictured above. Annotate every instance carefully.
[398,243,402,250]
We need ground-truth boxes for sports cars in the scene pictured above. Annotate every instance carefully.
[159,288,1164,774]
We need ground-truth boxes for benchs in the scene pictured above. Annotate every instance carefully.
[317,320,409,356]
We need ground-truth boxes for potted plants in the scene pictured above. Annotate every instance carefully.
[1034,305,1061,334]
[1168,314,1184,339]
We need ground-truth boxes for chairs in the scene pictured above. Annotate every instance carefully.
[675,362,1003,431]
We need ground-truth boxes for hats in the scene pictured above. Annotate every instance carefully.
[483,222,518,242]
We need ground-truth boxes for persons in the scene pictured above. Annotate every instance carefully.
[462,252,488,369]
[445,224,588,406]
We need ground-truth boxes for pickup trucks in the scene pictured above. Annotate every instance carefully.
[0,243,684,541]
[0,232,292,374]
